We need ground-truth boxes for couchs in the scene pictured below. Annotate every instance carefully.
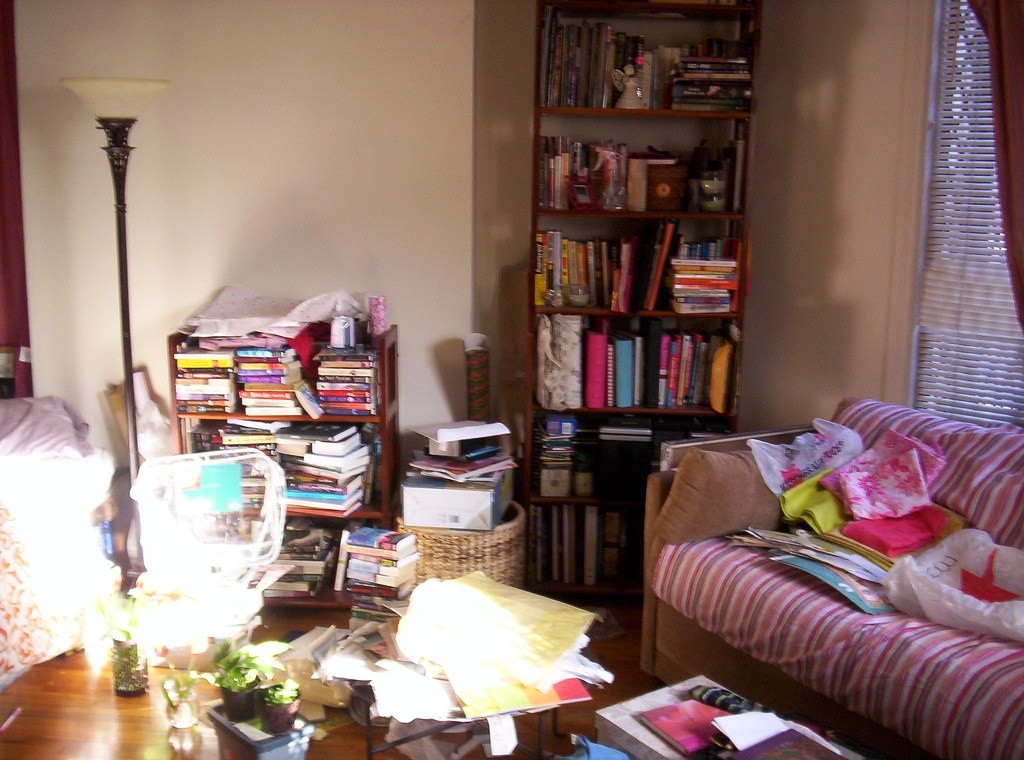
[638,389,1024,760]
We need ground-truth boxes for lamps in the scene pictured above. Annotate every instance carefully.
[68,79,171,480]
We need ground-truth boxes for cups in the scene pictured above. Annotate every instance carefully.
[330,317,355,348]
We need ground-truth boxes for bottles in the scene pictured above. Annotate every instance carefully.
[700,171,726,212]
[573,453,594,497]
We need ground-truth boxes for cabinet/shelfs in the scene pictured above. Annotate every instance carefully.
[168,322,399,609]
[530,1,759,602]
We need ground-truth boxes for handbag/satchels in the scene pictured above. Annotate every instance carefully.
[747,418,865,498]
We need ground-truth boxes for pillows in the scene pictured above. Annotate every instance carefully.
[654,449,781,544]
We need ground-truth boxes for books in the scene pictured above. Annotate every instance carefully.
[347,528,419,624]
[173,326,380,596]
[407,419,517,485]
[533,24,751,588]
[639,699,736,756]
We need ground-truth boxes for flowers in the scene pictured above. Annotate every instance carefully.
[270,679,299,703]
[213,639,293,693]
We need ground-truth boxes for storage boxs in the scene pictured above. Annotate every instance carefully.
[404,466,512,532]
[593,675,756,760]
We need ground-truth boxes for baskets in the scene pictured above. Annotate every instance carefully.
[393,500,525,591]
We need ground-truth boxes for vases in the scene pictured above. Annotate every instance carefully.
[219,686,255,721]
[263,687,302,736]
